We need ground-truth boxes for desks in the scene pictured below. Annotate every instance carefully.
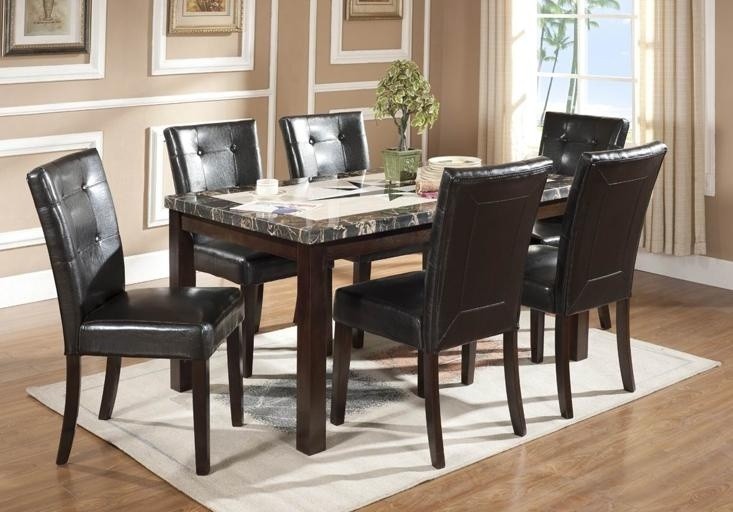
[165,169,589,455]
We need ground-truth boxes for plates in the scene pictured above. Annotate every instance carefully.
[246,189,287,202]
[413,154,485,181]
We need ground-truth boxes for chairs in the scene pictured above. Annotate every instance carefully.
[329,155,554,469]
[27,148,245,474]
[278,111,430,350]
[461,140,667,418]
[529,111,630,363]
[164,118,334,376]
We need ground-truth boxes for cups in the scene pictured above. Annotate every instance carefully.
[254,177,279,195]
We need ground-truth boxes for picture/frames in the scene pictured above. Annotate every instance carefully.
[345,0,403,21]
[168,0,242,34]
[1,0,92,57]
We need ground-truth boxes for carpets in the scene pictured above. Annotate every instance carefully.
[26,308,722,512]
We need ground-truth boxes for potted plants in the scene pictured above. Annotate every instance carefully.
[376,60,440,181]
